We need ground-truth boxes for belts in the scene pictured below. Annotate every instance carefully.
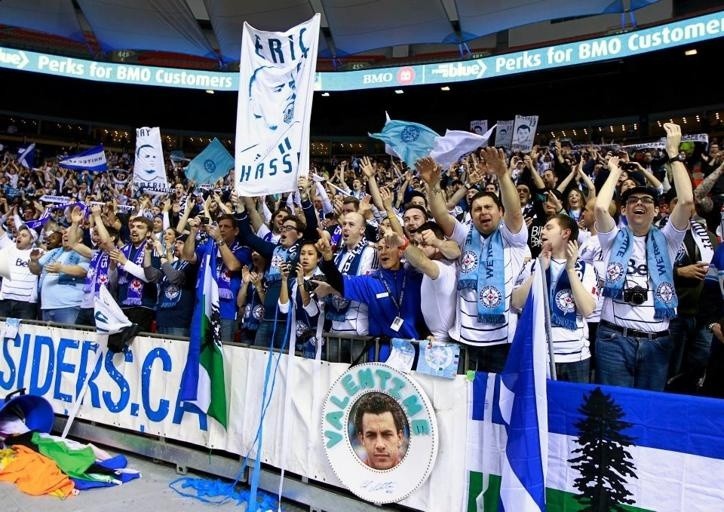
[600,319,670,341]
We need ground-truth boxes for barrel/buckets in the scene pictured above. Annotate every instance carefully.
[0,389,54,434]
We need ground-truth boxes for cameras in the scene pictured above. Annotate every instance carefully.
[623,285,648,304]
[145,243,156,249]
[286,259,299,277]
[200,217,210,224]
[621,162,638,171]
[534,193,549,203]
[305,275,327,292]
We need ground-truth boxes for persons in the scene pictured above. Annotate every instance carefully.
[354,393,406,470]
[0,122,724,391]
[248,65,297,131]
[0,402,31,441]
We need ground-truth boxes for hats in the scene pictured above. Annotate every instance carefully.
[19,225,38,244]
[621,185,659,207]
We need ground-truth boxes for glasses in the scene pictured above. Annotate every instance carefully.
[628,195,654,206]
